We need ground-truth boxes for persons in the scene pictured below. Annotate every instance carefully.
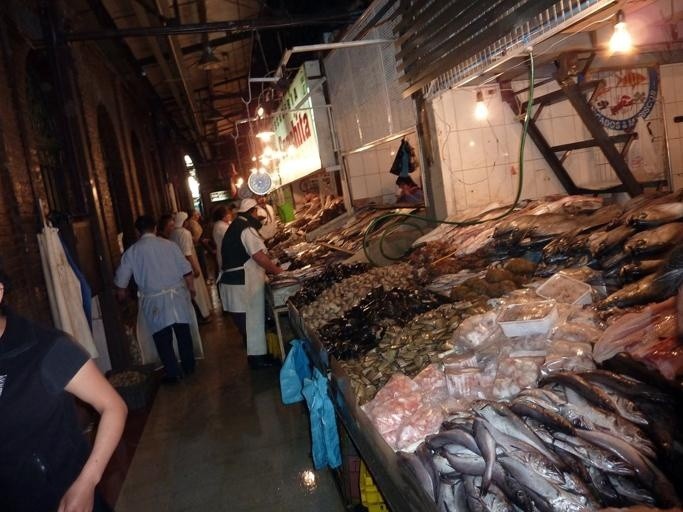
[115,194,286,387]
[0,267,129,512]
[396,176,424,202]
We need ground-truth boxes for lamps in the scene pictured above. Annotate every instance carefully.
[472,88,489,121]
[198,35,222,70]
[608,9,632,52]
[256,87,270,116]
[255,113,275,142]
[207,100,224,120]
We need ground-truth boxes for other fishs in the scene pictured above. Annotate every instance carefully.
[394,351,682,511]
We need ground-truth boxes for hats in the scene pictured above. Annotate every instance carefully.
[172,211,189,228]
[231,198,258,214]
